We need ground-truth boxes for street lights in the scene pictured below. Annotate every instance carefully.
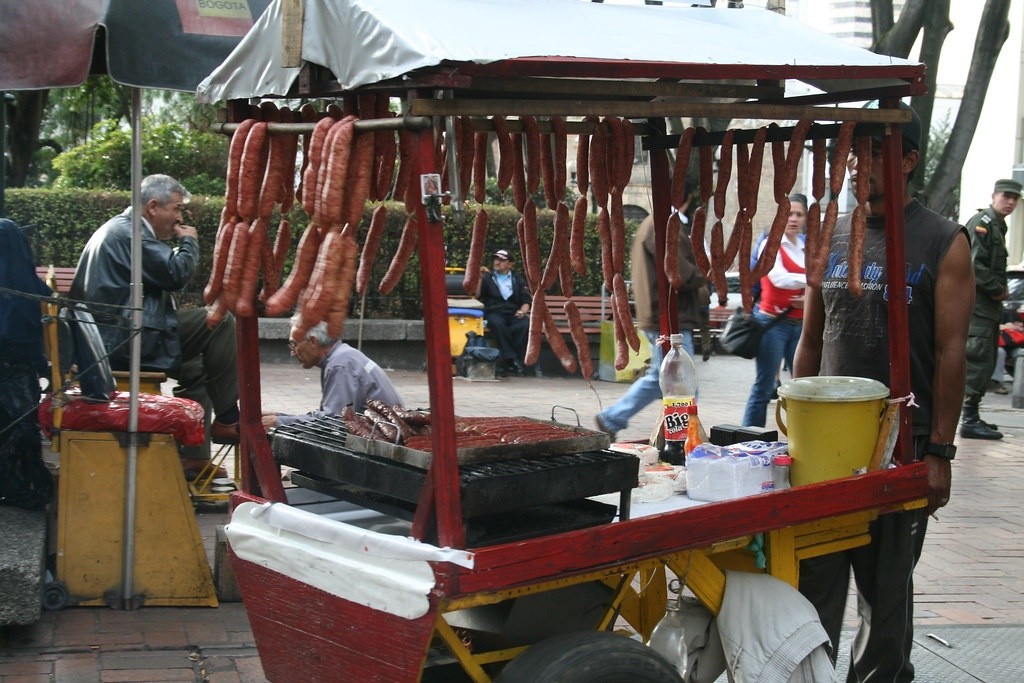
[567,158,598,215]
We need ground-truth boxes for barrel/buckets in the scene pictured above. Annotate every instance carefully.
[775,375,891,491]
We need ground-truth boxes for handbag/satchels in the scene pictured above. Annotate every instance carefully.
[719,309,763,359]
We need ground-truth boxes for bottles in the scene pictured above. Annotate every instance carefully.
[658,334,699,465]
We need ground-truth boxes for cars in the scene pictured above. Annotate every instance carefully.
[708,271,744,310]
[998,264,1024,348]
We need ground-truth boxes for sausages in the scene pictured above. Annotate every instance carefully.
[342,398,584,452]
[201,102,877,379]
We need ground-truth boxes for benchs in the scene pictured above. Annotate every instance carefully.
[529,295,613,336]
[36,266,77,293]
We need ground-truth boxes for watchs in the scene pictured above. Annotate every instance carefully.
[925,441,957,460]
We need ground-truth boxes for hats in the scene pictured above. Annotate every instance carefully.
[493,250,515,262]
[995,179,1023,198]
[859,99,922,149]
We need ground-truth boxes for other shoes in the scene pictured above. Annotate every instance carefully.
[180,456,227,480]
[212,418,242,445]
[507,359,526,377]
[595,415,616,443]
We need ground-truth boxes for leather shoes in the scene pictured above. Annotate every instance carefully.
[959,423,1004,440]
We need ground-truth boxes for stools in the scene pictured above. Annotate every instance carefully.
[110,368,161,399]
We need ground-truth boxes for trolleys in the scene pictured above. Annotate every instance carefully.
[190,0,934,683]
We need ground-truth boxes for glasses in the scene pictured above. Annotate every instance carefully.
[288,336,312,352]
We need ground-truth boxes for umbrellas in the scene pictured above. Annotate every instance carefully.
[0,0,276,610]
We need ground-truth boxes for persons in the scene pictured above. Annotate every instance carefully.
[959,178,1023,440]
[260,318,403,433]
[474,249,532,377]
[590,168,715,443]
[67,171,270,476]
[793,98,976,683]
[0,217,62,510]
[739,192,810,435]
